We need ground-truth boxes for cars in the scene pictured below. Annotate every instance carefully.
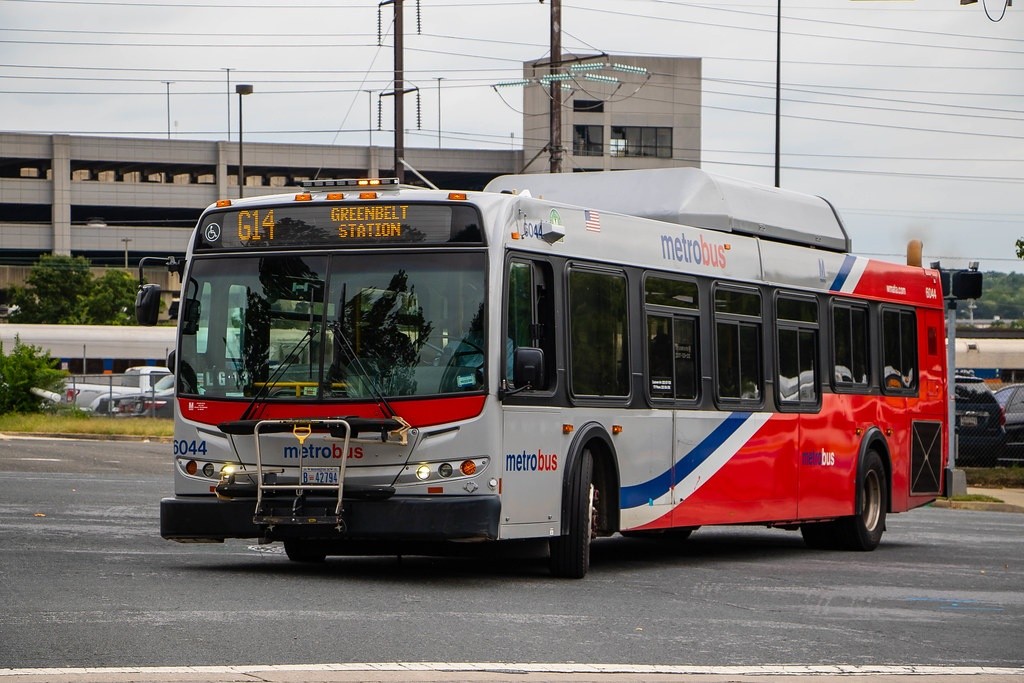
[993,383,1024,459]
[954,376,1006,466]
[99,375,174,418]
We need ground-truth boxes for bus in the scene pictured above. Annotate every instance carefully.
[135,167,954,580]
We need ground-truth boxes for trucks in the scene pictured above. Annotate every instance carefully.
[50,367,169,414]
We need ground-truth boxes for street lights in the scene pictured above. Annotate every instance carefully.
[161,82,175,139]
[235,84,253,199]
[365,90,378,146]
[221,68,235,143]
[433,77,446,148]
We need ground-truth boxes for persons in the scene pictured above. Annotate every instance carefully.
[435,303,514,384]
